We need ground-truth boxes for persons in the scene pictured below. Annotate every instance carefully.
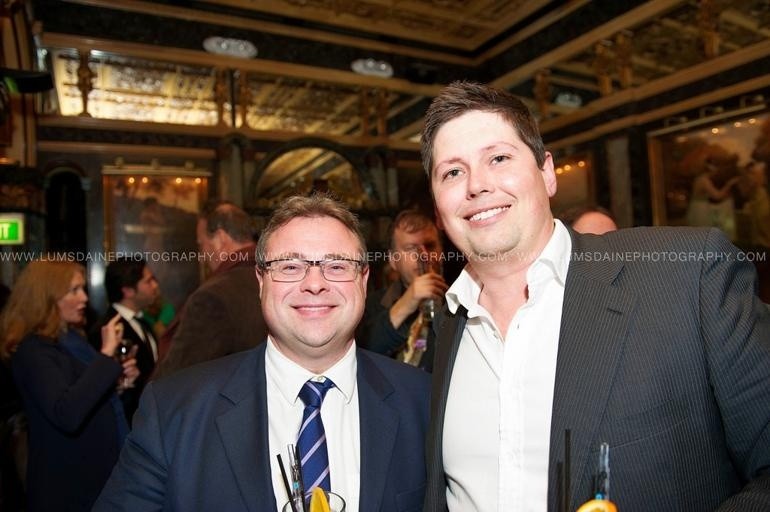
[358,209,455,374]
[93,257,166,431]
[0,254,139,511]
[141,294,174,340]
[562,203,620,237]
[733,162,769,244]
[157,203,268,378]
[138,195,166,255]
[87,188,437,512]
[420,81,770,512]
[686,142,741,244]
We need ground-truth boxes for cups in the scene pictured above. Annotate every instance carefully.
[282,492,347,512]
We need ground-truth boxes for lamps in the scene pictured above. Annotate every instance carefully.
[350,48,399,80]
[201,23,262,61]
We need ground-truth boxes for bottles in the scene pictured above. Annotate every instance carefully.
[417,260,443,321]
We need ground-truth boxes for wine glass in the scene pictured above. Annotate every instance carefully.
[113,339,135,390]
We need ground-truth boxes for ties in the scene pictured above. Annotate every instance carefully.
[295,378,335,510]
[132,316,154,362]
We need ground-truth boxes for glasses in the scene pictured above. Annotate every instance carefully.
[258,257,362,282]
